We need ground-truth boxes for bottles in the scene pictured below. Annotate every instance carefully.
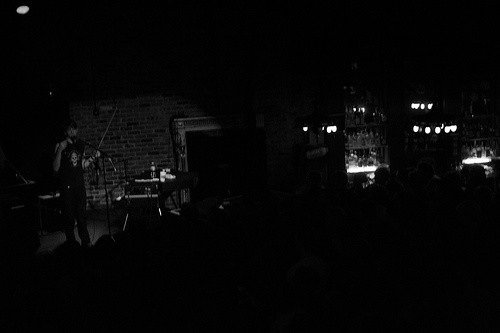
[345,98,389,175]
[460,90,500,163]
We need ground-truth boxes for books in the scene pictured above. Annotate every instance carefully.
[6,169,37,188]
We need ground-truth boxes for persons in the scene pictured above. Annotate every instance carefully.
[52,120,102,246]
[1,156,500,333]
